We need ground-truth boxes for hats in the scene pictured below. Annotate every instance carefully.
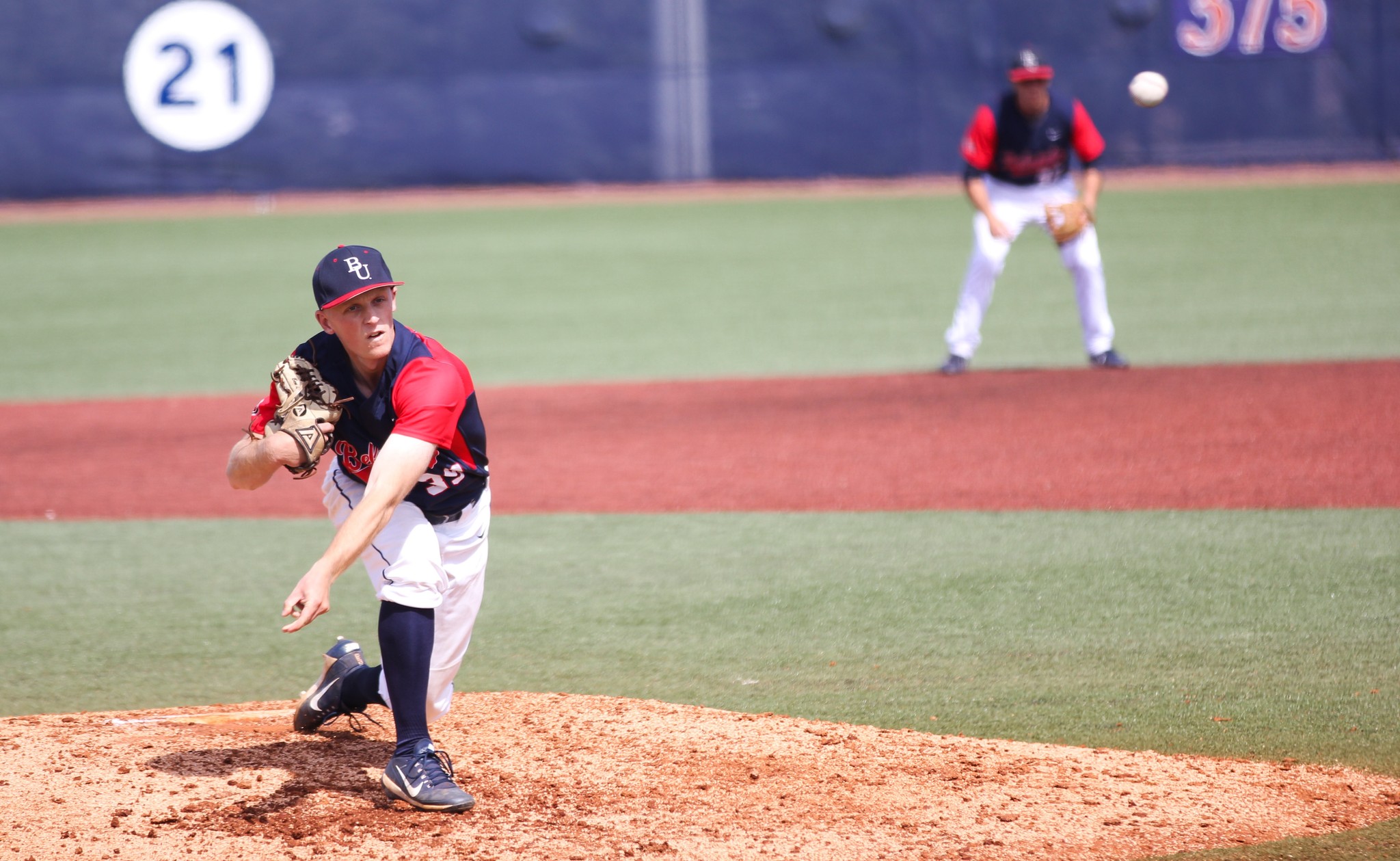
[313,244,404,308]
[1004,43,1056,83]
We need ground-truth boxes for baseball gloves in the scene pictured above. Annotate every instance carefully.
[1044,201,1089,245]
[269,355,353,479]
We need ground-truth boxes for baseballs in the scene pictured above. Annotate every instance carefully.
[1127,69,1171,108]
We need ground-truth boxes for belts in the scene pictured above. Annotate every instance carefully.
[425,481,489,525]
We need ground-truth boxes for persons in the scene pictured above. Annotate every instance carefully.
[227,245,490,814]
[940,47,1130,370]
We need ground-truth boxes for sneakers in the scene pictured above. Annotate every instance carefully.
[292,639,361,739]
[377,736,479,817]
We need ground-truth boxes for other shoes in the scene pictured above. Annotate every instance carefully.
[939,354,964,377]
[1089,349,1128,373]
